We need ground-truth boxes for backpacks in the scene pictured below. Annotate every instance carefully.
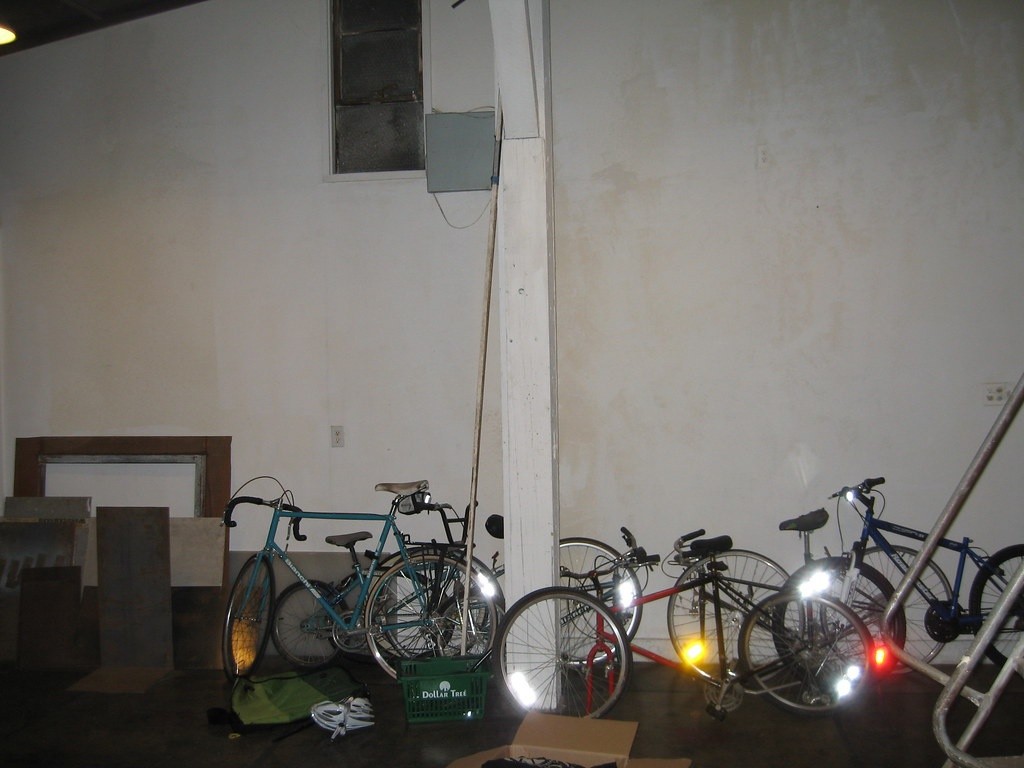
[205,663,369,738]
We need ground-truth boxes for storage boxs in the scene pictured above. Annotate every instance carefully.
[445,712,691,768]
[397,656,491,723]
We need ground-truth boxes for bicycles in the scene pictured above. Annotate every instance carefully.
[269,477,1023,744]
[220,475,506,690]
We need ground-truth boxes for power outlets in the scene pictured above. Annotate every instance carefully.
[331,426,345,447]
[982,383,1015,406]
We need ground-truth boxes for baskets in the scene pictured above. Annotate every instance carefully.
[396,657,495,724]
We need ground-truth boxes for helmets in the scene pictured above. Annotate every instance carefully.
[310,695,377,743]
